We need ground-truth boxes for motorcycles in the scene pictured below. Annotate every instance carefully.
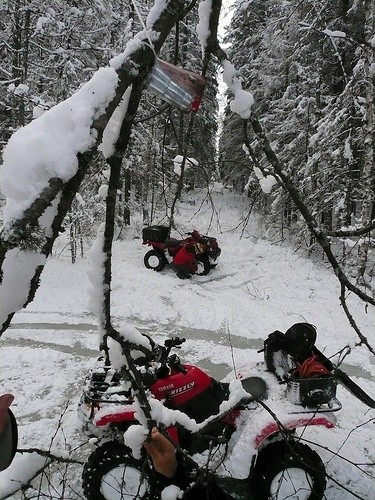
[141,226,218,276]
[78,323,343,500]
